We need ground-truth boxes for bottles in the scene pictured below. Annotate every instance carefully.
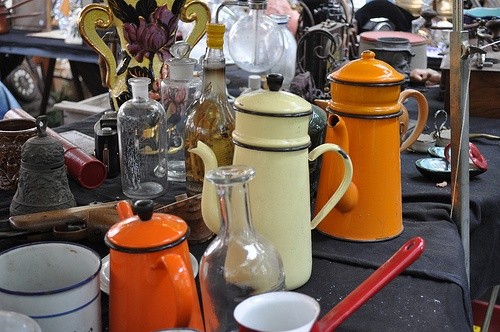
[200,165,287,332]
[184,23,236,195]
[241,76,264,96]
[265,15,298,89]
[157,57,203,182]
[117,77,167,200]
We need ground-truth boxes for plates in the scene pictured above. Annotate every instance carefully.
[100,252,197,295]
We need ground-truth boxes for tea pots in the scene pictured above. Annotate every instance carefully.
[314,51,428,242]
[106,200,204,332]
[189,74,353,290]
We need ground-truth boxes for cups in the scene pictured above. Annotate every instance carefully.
[0,119,38,192]
[0,310,42,332]
[234,292,320,332]
[0,242,103,332]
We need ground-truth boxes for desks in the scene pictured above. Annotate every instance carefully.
[1,64,500,332]
[0,26,100,120]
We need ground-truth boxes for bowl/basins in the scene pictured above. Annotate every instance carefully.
[436,130,451,147]
[416,157,485,180]
[428,147,451,159]
[411,134,436,152]
